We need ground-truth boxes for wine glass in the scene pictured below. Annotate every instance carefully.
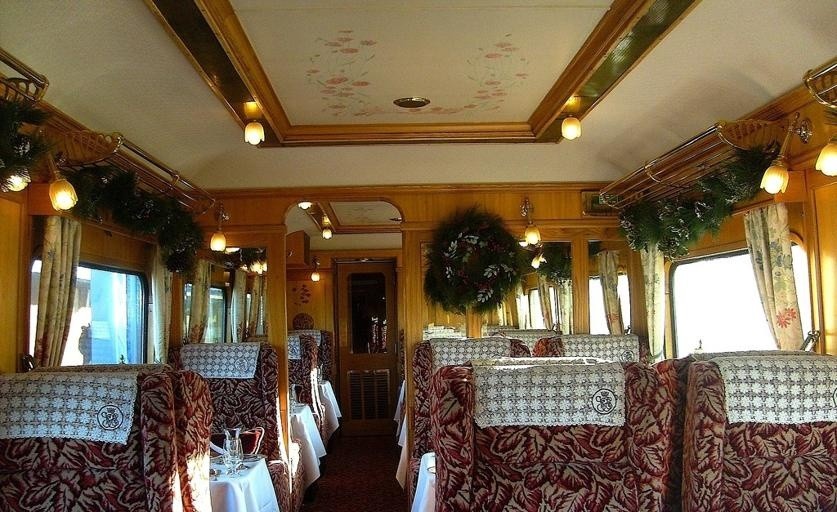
[224,438,245,478]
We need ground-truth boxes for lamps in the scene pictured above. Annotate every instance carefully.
[518,195,541,245]
[531,250,547,269]
[250,258,267,274]
[310,256,320,282]
[243,120,266,146]
[36,128,80,212]
[322,227,332,241]
[759,113,810,193]
[210,205,229,252]
[562,93,582,114]
[816,130,837,178]
[243,101,263,122]
[561,115,582,141]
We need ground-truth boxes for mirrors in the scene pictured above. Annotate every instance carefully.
[182,248,267,343]
[588,239,631,335]
[498,243,574,333]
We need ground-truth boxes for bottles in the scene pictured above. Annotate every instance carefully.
[221,427,246,472]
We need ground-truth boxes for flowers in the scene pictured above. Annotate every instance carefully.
[424,205,527,315]
